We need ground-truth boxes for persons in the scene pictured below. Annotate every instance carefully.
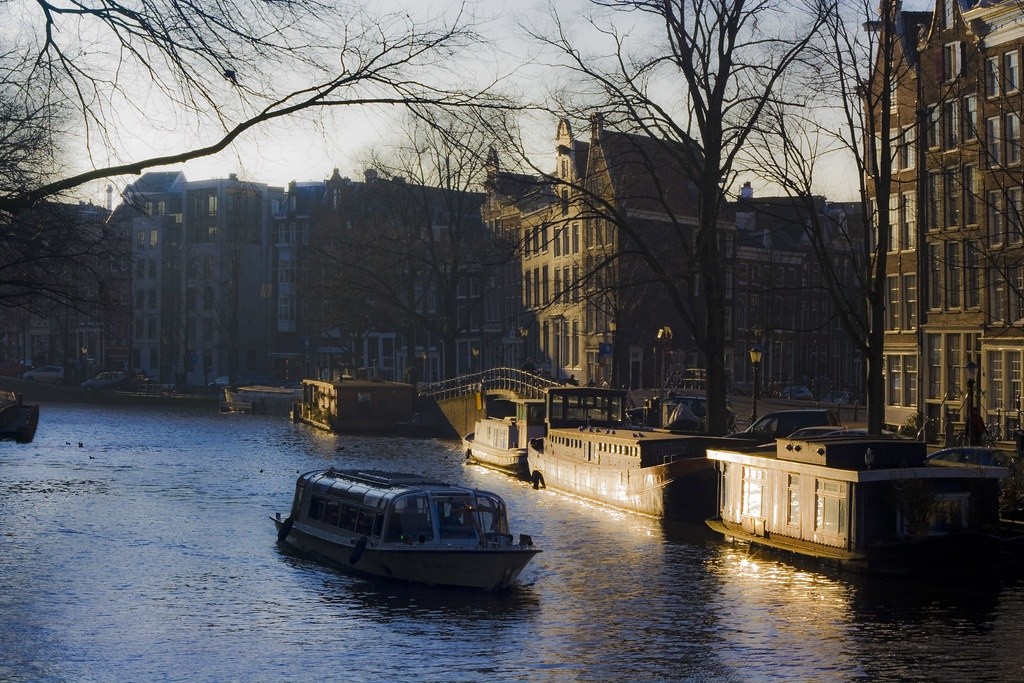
[600,376,608,389]
[518,357,538,388]
[561,374,579,386]
[965,407,990,446]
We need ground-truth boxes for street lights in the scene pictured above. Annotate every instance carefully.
[519,325,528,363]
[962,361,979,446]
[608,319,617,388]
[81,346,88,377]
[472,345,480,374]
[422,351,430,382]
[748,345,764,425]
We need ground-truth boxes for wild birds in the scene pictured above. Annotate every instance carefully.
[632,432,647,439]
[577,424,616,435]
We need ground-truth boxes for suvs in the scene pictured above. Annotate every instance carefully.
[625,397,736,428]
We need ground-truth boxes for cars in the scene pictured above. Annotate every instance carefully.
[23,366,64,385]
[925,446,1004,467]
[81,372,126,392]
[723,409,841,443]
[755,425,910,449]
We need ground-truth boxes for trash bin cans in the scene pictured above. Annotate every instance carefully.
[643,399,659,428]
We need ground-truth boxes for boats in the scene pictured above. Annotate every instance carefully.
[527,386,718,522]
[218,380,301,415]
[269,465,544,587]
[460,399,593,479]
